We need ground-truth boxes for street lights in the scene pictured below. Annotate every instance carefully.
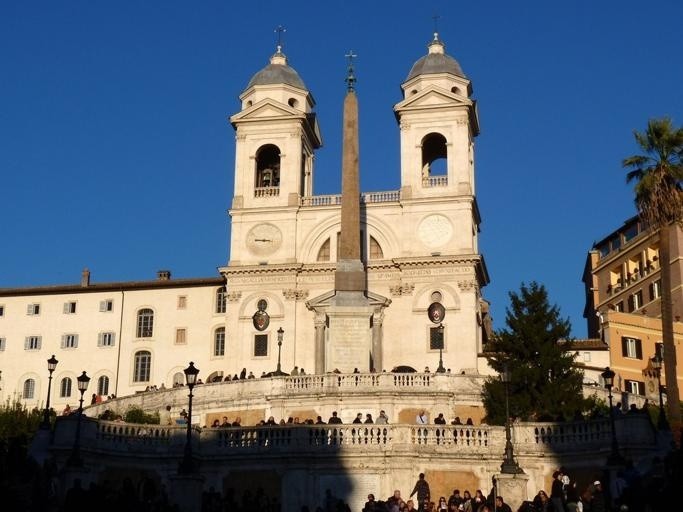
[599,366,625,467]
[169,358,205,473]
[273,326,283,375]
[435,324,445,373]
[29,355,58,429]
[61,371,90,469]
[648,353,675,455]
[492,363,529,475]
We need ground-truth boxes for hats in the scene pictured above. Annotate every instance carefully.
[593,480,600,485]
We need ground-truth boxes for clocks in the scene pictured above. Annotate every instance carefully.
[243,221,283,259]
